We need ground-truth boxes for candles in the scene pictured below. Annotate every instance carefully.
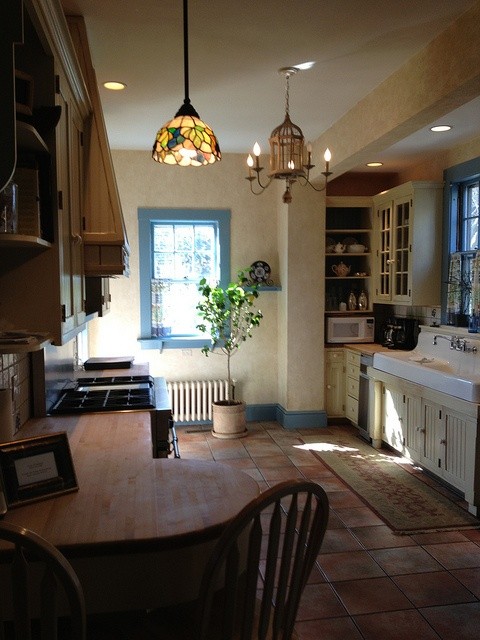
[246,154,254,178]
[323,146,332,172]
[307,141,312,164]
[252,141,261,167]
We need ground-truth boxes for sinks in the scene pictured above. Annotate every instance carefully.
[373,351,480,402]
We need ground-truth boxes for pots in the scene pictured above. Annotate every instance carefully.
[347,243,368,253]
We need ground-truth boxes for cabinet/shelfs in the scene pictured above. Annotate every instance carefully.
[240,286,280,293]
[325,195,374,313]
[374,180,445,307]
[443,393,480,506]
[86,37,132,277]
[345,347,361,425]
[0,37,63,353]
[404,380,442,478]
[324,347,346,418]
[84,276,112,322]
[51,37,87,347]
[381,372,403,455]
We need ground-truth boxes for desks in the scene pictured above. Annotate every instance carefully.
[0,413,260,639]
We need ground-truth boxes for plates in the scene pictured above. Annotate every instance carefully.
[249,260,271,282]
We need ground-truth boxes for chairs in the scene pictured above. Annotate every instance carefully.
[0,521,86,639]
[194,476,330,640]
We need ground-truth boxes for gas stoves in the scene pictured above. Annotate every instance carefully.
[50,375,156,416]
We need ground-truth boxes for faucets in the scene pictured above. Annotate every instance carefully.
[432,335,462,350]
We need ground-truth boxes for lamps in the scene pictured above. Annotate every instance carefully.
[151,0,223,168]
[244,67,333,205]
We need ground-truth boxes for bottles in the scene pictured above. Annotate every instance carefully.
[0,178,19,234]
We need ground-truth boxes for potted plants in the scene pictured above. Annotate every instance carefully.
[195,267,264,440]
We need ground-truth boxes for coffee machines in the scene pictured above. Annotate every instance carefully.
[382,317,418,350]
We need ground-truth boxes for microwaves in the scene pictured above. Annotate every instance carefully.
[327,316,376,345]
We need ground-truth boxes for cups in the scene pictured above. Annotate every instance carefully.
[340,303,347,311]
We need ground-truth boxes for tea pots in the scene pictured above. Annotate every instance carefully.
[331,261,353,277]
[333,242,347,255]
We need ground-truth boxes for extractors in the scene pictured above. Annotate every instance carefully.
[82,244,133,279]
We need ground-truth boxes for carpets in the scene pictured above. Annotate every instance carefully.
[296,434,480,536]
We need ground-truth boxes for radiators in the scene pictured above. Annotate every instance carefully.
[167,378,238,426]
